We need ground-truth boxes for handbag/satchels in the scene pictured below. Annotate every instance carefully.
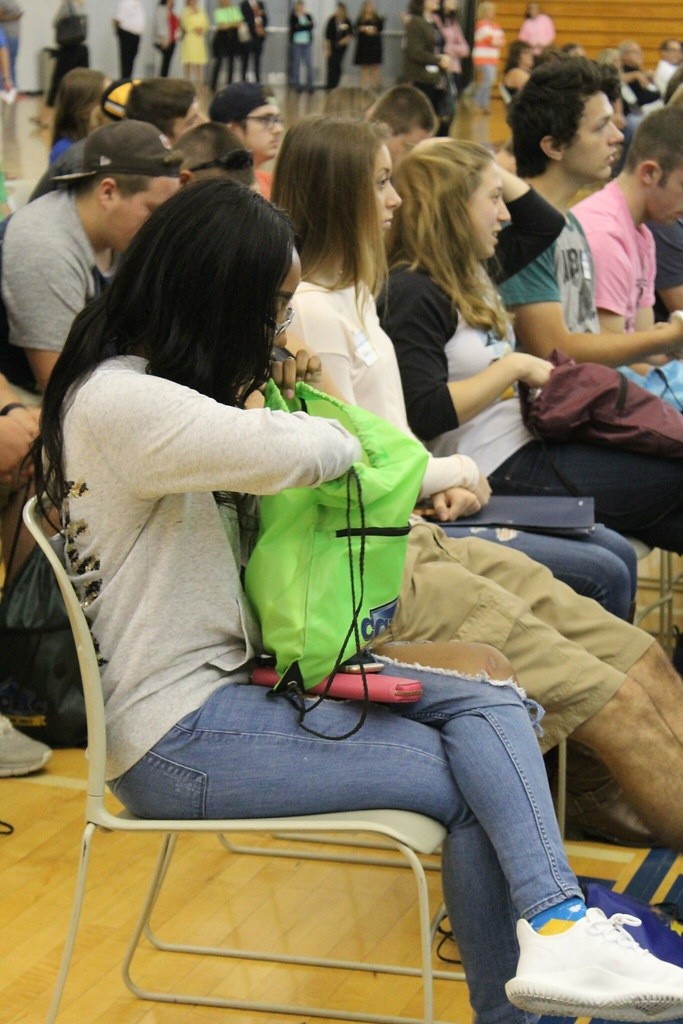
[0,471,87,747]
[243,375,429,743]
[526,347,683,475]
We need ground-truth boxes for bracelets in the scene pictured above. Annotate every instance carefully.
[0,403,27,417]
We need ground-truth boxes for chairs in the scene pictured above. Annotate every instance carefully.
[22,492,683,1024]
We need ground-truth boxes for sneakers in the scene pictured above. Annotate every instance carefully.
[505,908,683,1022]
[0,716,52,777]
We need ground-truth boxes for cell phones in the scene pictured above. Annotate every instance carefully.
[339,662,387,674]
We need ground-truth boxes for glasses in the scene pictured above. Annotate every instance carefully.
[189,150,254,173]
[246,114,284,127]
[264,307,297,335]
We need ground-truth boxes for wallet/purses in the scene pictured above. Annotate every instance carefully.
[251,667,423,703]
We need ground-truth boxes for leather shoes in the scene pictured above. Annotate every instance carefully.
[547,776,664,846]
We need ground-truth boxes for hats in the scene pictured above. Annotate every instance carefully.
[51,120,182,181]
[209,83,271,123]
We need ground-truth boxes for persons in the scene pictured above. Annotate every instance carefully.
[17,176,683,1024]
[0,0,682,848]
